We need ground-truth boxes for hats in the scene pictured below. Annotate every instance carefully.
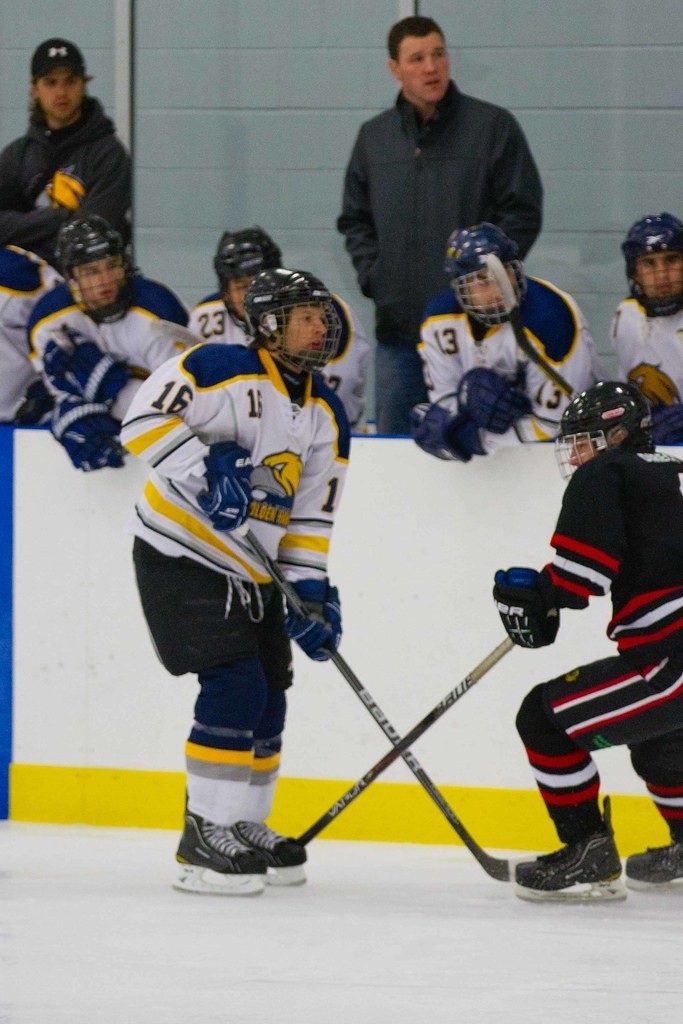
[32,37,84,84]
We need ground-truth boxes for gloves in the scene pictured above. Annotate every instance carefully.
[455,369,530,433]
[195,441,252,529]
[284,576,343,664]
[50,401,125,471]
[41,322,130,412]
[492,566,559,648]
[411,400,480,464]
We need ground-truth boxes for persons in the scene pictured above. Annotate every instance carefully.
[187,229,365,435]
[408,223,602,464]
[0,37,133,428]
[494,381,683,900]
[613,210,683,443]
[26,216,192,473]
[121,268,351,895]
[337,16,544,434]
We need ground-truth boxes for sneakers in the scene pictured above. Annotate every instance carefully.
[231,820,307,884]
[171,791,270,894]
[624,841,683,887]
[515,796,627,901]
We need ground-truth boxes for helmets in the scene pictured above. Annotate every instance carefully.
[211,224,282,329]
[445,222,528,325]
[244,271,340,375]
[621,213,683,316]
[57,214,137,323]
[554,380,653,485]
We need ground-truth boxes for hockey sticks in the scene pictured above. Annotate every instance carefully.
[297,636,515,847]
[510,305,582,401]
[237,522,534,882]
